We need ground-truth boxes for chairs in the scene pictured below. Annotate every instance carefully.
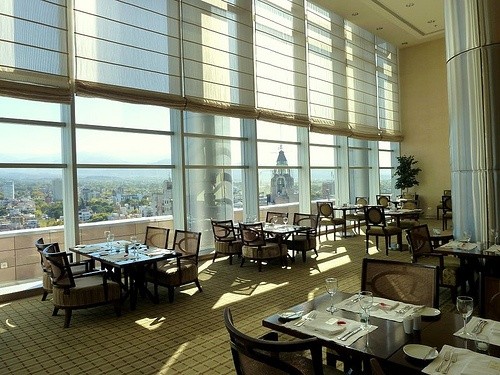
[36,190,500,375]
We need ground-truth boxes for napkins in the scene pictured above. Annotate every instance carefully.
[100,253,135,262]
[280,310,379,346]
[453,316,500,347]
[421,344,500,375]
[333,294,422,322]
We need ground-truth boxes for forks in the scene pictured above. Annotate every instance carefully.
[436,350,458,374]
[293,312,318,327]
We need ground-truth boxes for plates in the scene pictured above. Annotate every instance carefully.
[421,307,441,317]
[403,344,439,359]
[277,310,302,319]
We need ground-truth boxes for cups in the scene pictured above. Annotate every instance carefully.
[477,241,483,251]
[475,333,490,351]
[403,313,421,334]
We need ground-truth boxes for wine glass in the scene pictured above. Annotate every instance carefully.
[273,216,288,228]
[325,278,338,312]
[463,231,472,250]
[398,202,403,212]
[456,296,473,327]
[130,235,137,253]
[104,229,114,253]
[358,291,373,328]
[490,228,499,245]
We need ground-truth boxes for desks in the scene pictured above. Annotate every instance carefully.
[333,207,363,237]
[385,211,424,251]
[430,231,453,249]
[226,222,313,262]
[387,312,500,375]
[69,240,182,311]
[262,291,443,375]
[434,241,500,318]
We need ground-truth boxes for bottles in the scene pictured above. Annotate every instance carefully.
[125,242,128,253]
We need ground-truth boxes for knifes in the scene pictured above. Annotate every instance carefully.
[422,346,437,361]
[339,326,362,341]
